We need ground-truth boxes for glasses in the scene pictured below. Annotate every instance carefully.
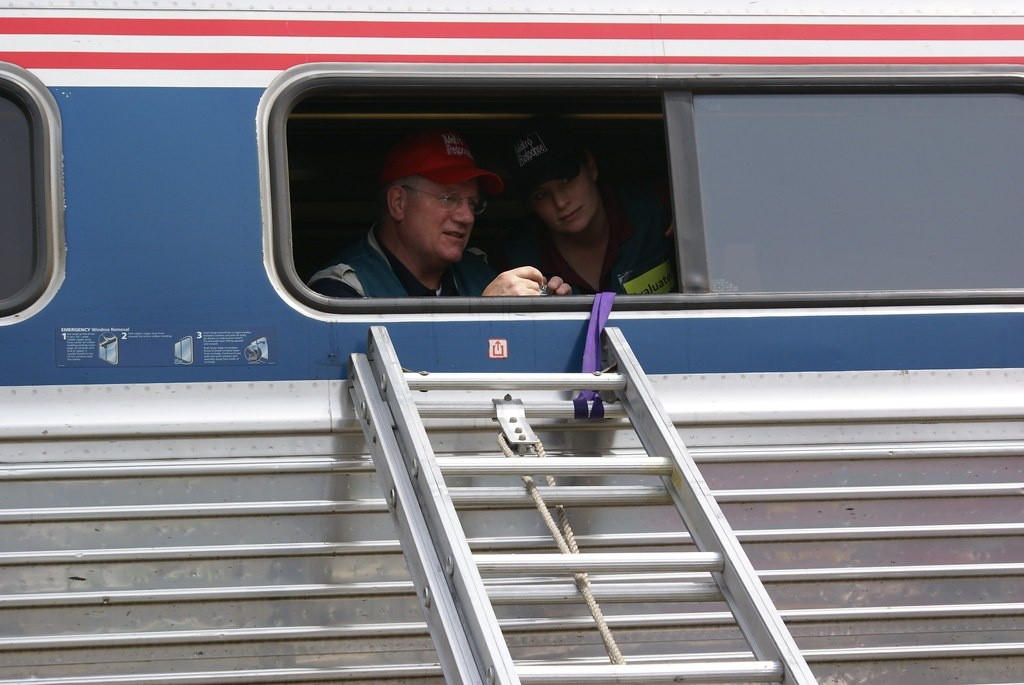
[400,184,487,215]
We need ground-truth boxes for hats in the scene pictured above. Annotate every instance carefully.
[379,127,505,196]
[497,114,581,200]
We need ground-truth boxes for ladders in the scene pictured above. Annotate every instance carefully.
[346,323,818,685]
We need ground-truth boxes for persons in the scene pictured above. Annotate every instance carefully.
[307,125,573,298]
[491,111,678,294]
